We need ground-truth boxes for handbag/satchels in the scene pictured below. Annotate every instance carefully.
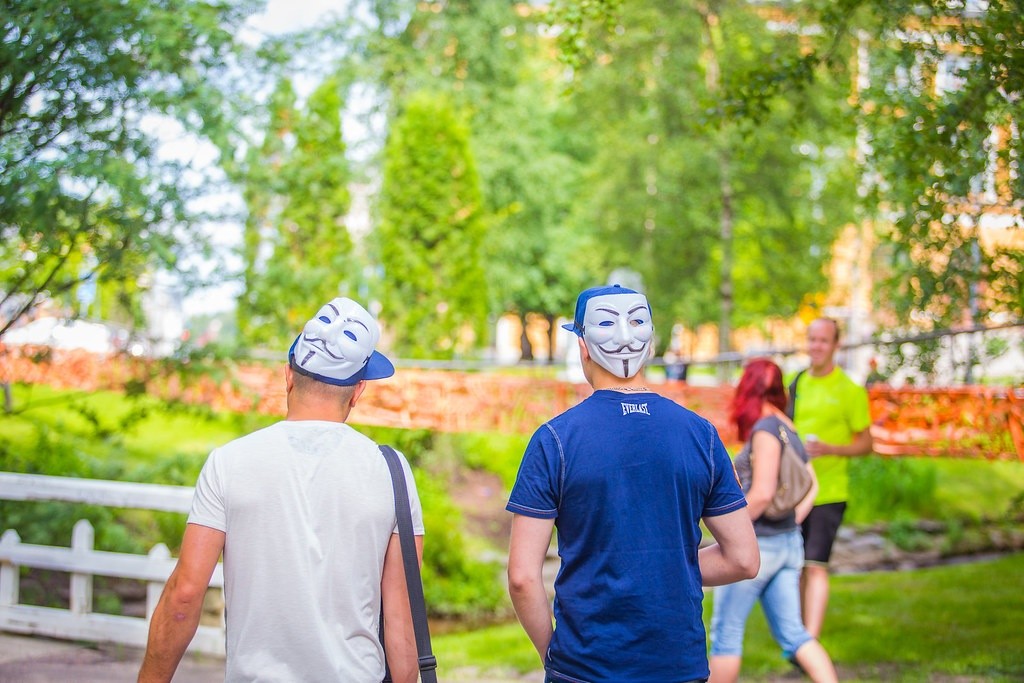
[747,415,813,521]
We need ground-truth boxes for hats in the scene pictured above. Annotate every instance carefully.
[561,283,655,340]
[288,297,395,387]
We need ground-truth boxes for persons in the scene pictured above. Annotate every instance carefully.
[900,377,916,390]
[508,287,760,683]
[866,361,889,389]
[133,299,425,683]
[782,317,870,683]
[708,355,838,683]
